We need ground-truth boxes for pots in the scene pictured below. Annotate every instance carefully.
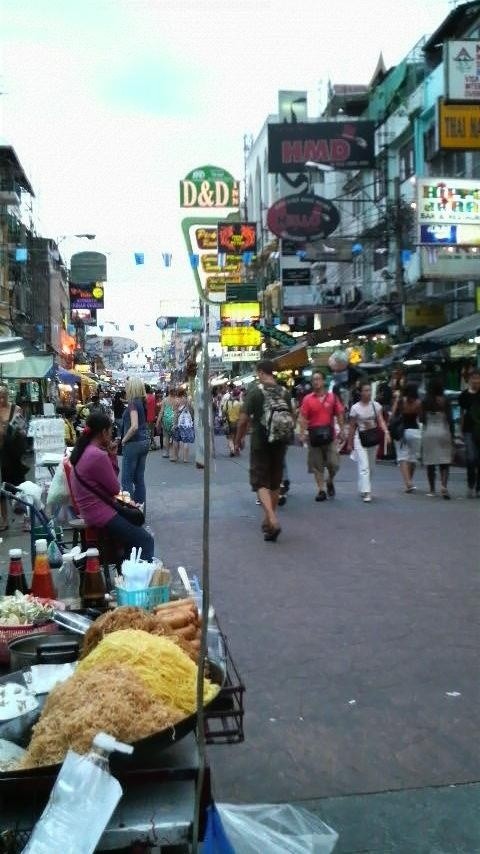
[1,658,227,791]
[8,631,91,673]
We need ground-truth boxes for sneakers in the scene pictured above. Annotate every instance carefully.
[264,523,281,542]
[262,521,271,532]
[277,494,287,506]
[327,483,334,496]
[363,492,371,502]
[441,487,451,499]
[315,491,327,501]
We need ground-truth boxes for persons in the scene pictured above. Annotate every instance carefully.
[87,390,127,456]
[219,383,244,456]
[456,367,480,497]
[298,371,346,502]
[70,413,154,562]
[387,378,456,499]
[347,383,390,501]
[0,384,26,529]
[234,362,296,540]
[144,383,194,464]
[120,375,150,516]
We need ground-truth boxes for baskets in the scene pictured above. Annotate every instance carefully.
[116,585,170,609]
[0,596,65,665]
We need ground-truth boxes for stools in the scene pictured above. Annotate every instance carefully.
[69,517,113,561]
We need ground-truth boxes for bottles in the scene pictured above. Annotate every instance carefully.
[108,589,119,610]
[33,537,56,600]
[18,710,134,854]
[84,546,107,609]
[58,544,81,611]
[28,412,67,483]
[5,547,31,595]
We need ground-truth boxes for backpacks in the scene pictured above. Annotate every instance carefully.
[257,383,295,444]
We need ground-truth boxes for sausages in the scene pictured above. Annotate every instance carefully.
[153,597,211,680]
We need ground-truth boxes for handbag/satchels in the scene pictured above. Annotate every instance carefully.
[308,426,335,448]
[359,427,385,447]
[112,498,145,527]
[388,412,404,441]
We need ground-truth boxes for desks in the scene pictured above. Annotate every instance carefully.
[33,456,61,478]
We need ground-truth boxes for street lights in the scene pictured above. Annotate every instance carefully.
[55,230,97,248]
